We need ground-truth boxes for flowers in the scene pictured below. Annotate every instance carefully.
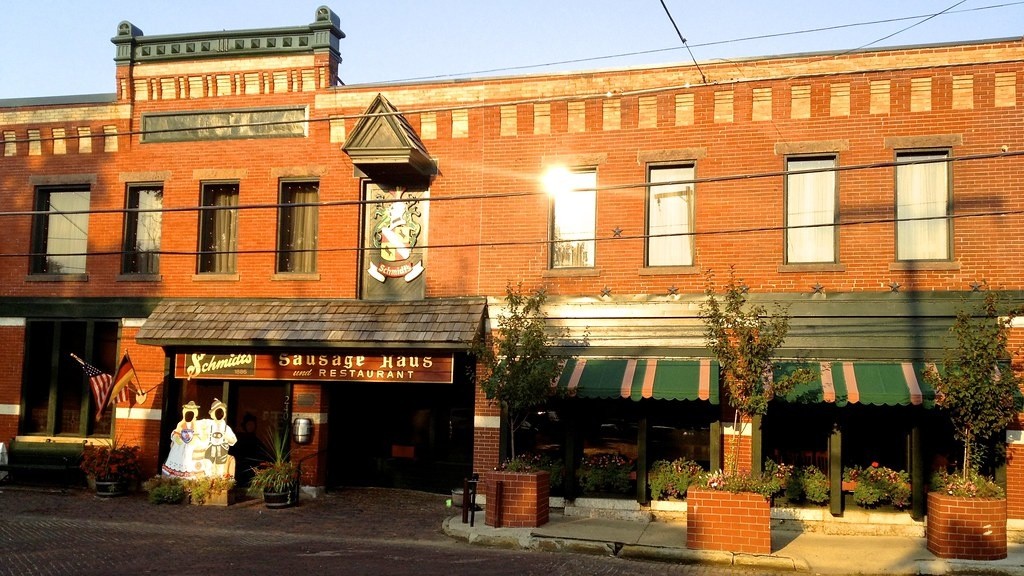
[79,427,147,482]
[245,422,305,499]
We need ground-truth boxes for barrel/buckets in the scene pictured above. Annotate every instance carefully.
[451,488,473,507]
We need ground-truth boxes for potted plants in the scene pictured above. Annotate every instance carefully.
[141,474,188,506]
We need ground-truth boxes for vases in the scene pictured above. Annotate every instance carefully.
[451,488,473,507]
[94,476,133,497]
[263,480,299,510]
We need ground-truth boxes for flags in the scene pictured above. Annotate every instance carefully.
[83,362,128,411]
[96,354,136,422]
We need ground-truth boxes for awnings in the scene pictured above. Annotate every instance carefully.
[496,358,720,404]
[773,361,1024,407]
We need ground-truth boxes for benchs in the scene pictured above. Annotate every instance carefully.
[0,437,88,490]
